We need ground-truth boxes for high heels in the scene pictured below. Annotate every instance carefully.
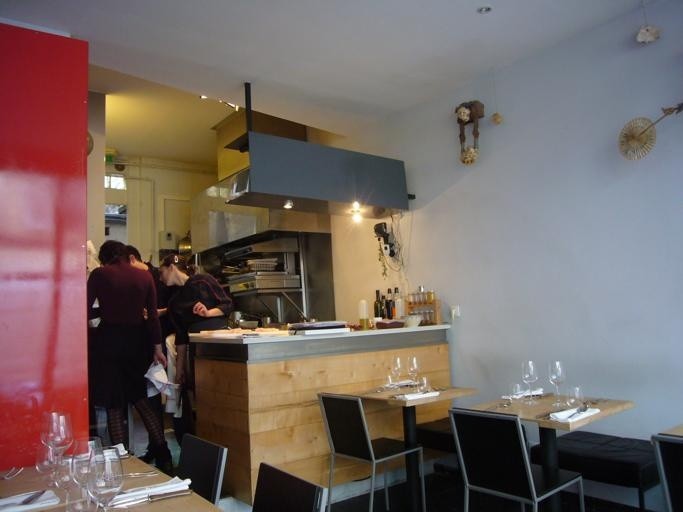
[156,442,174,472]
[138,439,155,463]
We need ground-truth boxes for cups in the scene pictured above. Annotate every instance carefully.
[565,384,584,409]
[417,376,431,393]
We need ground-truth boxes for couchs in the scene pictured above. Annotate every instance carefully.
[416,416,456,454]
[529,431,662,512]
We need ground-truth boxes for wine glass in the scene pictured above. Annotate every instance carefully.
[35,445,58,475]
[49,412,73,467]
[547,360,566,407]
[88,447,124,512]
[52,458,84,505]
[70,436,104,499]
[40,411,67,464]
[405,356,419,392]
[64,483,101,511]
[520,360,541,407]
[391,356,403,392]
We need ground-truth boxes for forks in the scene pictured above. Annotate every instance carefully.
[1,465,23,481]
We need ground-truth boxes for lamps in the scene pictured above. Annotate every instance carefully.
[105,171,128,191]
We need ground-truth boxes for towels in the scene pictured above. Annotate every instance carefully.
[110,476,189,508]
[0,490,59,512]
[112,443,128,456]
[398,391,440,400]
[550,406,600,423]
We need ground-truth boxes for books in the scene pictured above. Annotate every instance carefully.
[287,321,351,337]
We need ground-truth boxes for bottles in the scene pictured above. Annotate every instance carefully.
[373,289,384,318]
[386,287,397,320]
[406,285,440,325]
[394,287,405,319]
[379,295,390,319]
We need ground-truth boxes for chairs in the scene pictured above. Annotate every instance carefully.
[318,393,428,511]
[251,462,327,512]
[650,432,683,512]
[174,433,228,507]
[447,408,585,512]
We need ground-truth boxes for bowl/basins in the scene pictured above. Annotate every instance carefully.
[242,320,258,331]
[402,315,422,328]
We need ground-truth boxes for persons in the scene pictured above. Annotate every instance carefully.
[86,240,174,472]
[159,254,233,447]
[126,245,172,464]
